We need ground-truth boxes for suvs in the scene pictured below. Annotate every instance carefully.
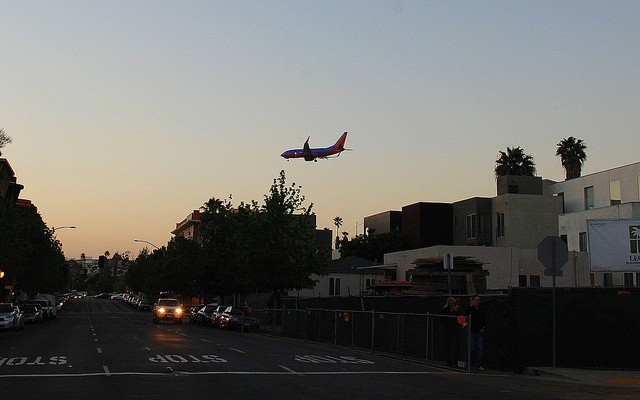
[20,304,43,323]
[220,306,251,330]
[31,300,57,320]
[152,298,182,324]
[0,303,24,329]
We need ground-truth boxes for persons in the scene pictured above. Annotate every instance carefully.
[442,297,465,368]
[464,295,490,370]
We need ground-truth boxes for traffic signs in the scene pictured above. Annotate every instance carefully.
[443,252,453,269]
[538,236,568,269]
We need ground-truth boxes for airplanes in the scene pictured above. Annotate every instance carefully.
[280,132,352,162]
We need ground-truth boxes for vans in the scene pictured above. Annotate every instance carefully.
[57,294,69,300]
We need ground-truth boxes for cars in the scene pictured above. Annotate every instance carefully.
[111,293,124,299]
[197,306,215,324]
[74,294,82,299]
[95,293,112,299]
[211,306,228,325]
[57,301,63,310]
[122,294,151,311]
